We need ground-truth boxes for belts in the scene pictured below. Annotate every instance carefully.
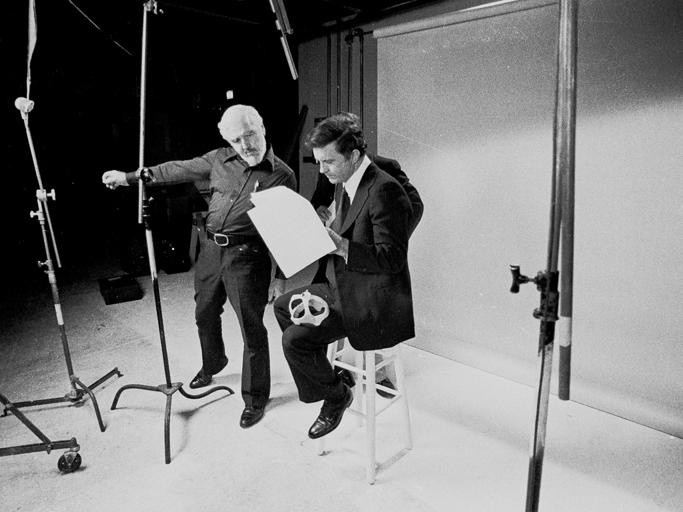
[207,232,245,246]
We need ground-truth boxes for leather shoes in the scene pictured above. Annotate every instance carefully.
[239,402,266,427]
[308,366,357,438]
[190,358,229,389]
[375,378,396,398]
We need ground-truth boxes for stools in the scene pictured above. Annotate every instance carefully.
[313,331,417,489]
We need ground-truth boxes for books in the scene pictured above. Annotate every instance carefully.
[246,185,338,278]
[325,200,337,229]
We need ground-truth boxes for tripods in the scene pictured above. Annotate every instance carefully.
[111,1,233,465]
[2,113,124,434]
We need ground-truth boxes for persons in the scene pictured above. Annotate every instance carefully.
[273,113,415,439]
[100,104,297,429]
[308,111,424,400]
